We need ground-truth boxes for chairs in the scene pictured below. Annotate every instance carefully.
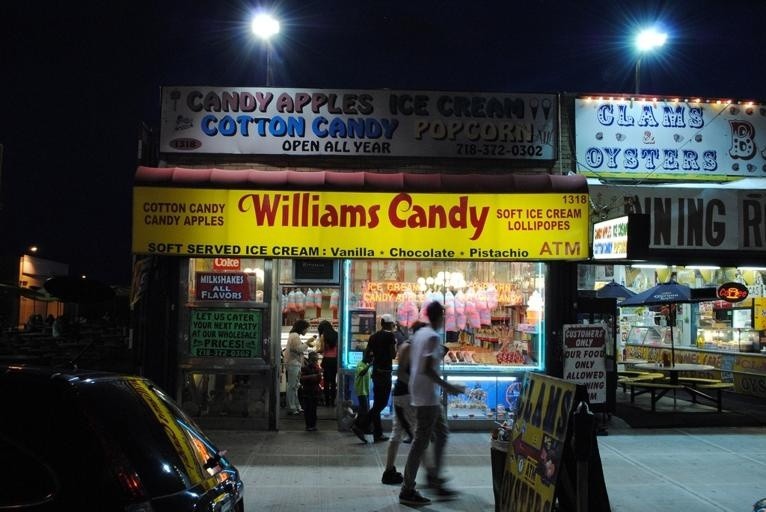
[352,426,413,444]
[397,477,461,506]
[381,470,404,484]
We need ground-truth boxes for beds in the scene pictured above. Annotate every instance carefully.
[587,275,720,367]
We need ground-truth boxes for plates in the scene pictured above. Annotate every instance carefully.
[626,326,662,347]
[696,326,760,353]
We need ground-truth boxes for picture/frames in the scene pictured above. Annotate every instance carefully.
[616,359,715,385]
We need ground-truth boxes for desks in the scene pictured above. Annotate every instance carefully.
[256,14,280,88]
[634,28,657,93]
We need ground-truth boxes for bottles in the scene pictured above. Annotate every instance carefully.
[381,313,398,325]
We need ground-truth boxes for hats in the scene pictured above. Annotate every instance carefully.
[0,362,244,512]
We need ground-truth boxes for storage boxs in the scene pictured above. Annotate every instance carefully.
[617,371,734,412]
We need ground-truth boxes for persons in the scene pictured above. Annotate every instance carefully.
[350,301,469,507]
[283,318,338,432]
